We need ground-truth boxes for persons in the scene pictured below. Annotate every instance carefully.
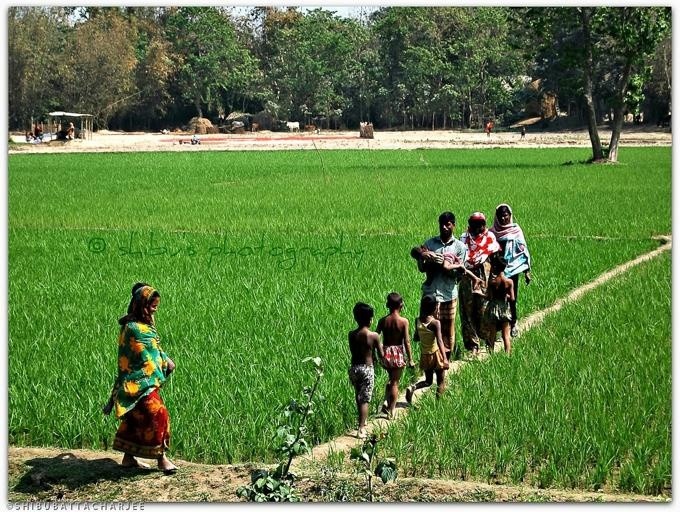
[487,203,533,338]
[348,301,385,439]
[458,211,499,358]
[373,292,416,422]
[410,246,482,290]
[485,256,516,356]
[417,210,469,359]
[109,284,179,473]
[405,294,452,410]
[25,118,75,145]
